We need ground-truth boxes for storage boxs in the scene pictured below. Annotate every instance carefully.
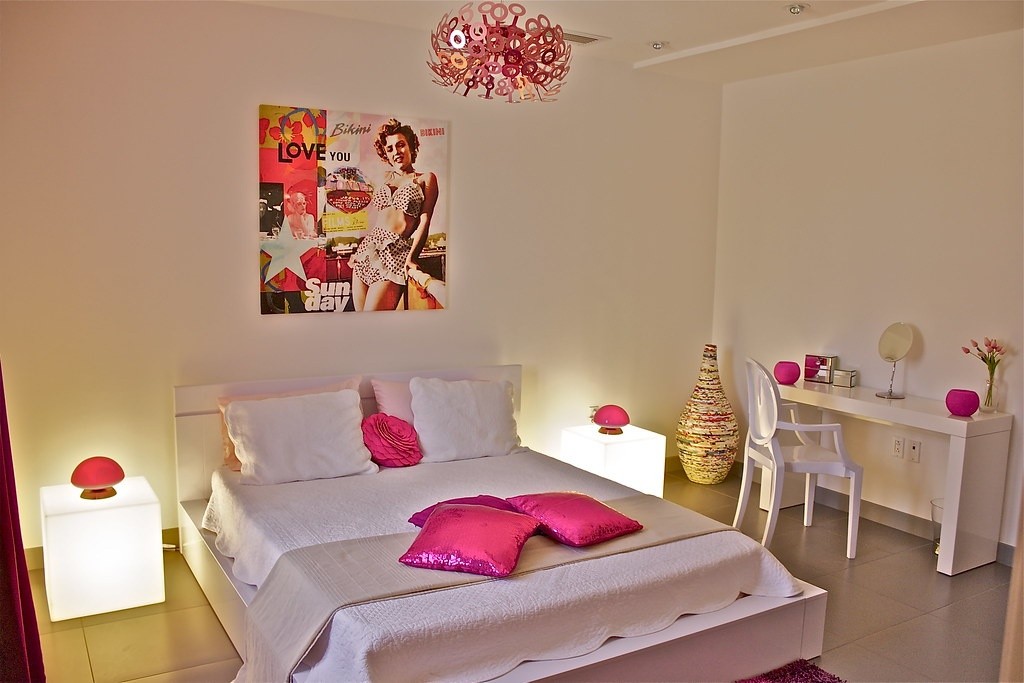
[804,354,835,383]
[833,369,856,387]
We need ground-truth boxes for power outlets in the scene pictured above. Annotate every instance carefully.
[893,437,904,458]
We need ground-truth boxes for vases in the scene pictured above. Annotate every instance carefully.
[674,344,738,484]
[979,380,999,412]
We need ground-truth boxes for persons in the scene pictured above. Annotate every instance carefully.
[346,119,439,312]
[287,192,314,239]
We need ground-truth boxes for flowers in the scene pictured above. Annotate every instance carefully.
[962,336,1008,406]
[360,412,424,467]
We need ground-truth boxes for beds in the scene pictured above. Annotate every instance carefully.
[172,364,830,683]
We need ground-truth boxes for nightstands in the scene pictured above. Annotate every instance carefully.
[548,420,667,500]
[39,478,167,623]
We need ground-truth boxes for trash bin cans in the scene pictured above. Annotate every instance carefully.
[930,498,945,554]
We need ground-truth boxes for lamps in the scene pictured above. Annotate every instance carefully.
[593,405,630,435]
[70,456,125,499]
[426,1,572,104]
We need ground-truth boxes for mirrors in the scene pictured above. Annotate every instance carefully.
[874,322,913,400]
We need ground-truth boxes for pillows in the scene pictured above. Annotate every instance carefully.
[503,490,643,546]
[407,494,519,529]
[407,373,526,462]
[218,389,380,484]
[218,373,359,473]
[401,505,542,577]
[371,381,414,429]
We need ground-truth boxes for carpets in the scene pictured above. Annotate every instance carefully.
[734,658,847,683]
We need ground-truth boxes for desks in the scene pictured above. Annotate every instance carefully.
[759,380,1014,575]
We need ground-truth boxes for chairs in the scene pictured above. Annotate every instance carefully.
[732,357,863,559]
